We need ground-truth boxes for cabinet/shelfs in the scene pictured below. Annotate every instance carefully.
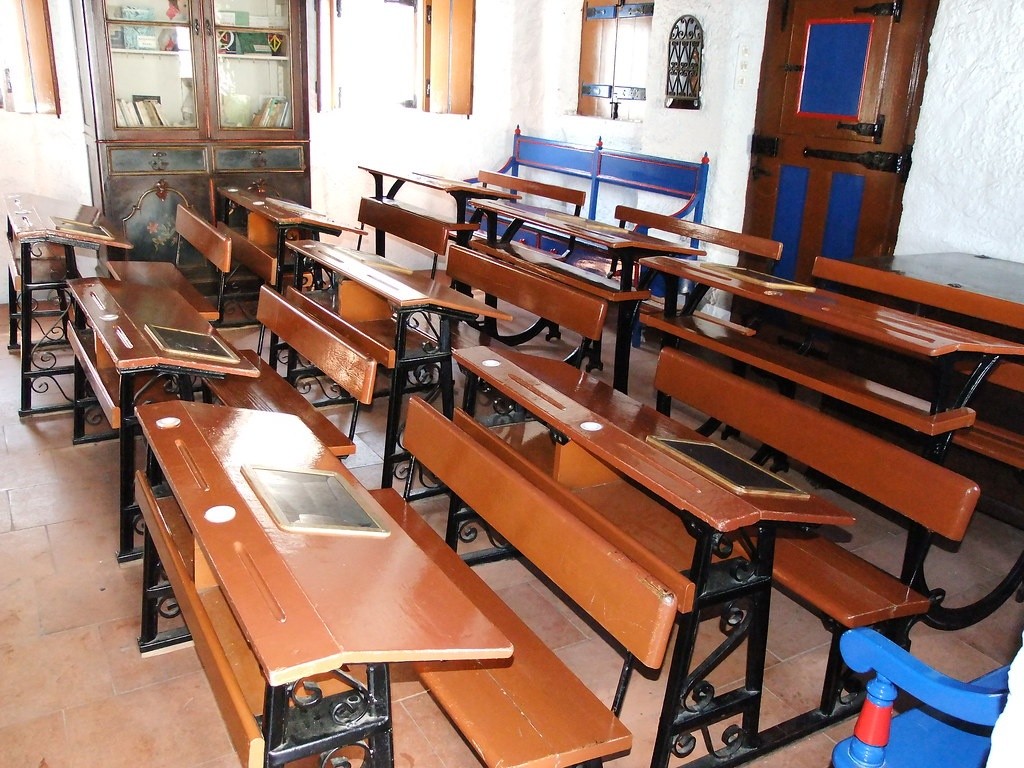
[70,0,313,302]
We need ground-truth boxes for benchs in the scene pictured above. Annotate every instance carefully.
[2,165,1024,768]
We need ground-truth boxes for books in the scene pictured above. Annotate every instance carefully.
[114,92,293,128]
[111,4,290,58]
